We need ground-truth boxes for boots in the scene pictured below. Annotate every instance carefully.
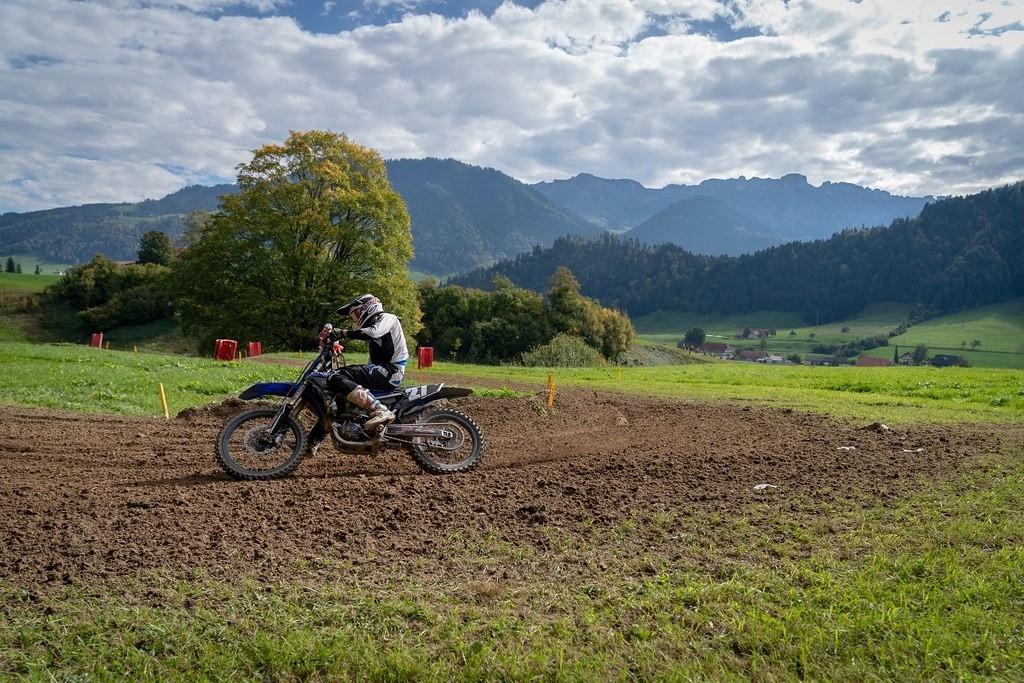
[282,420,331,460]
[346,384,395,430]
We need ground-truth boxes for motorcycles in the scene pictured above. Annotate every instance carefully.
[215,323,485,479]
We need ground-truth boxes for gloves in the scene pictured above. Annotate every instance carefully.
[318,327,344,348]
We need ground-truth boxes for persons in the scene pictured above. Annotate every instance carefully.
[319,324,339,349]
[326,294,409,431]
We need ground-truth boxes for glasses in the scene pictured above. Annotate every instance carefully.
[351,305,368,322]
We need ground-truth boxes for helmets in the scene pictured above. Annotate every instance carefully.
[336,294,384,330]
